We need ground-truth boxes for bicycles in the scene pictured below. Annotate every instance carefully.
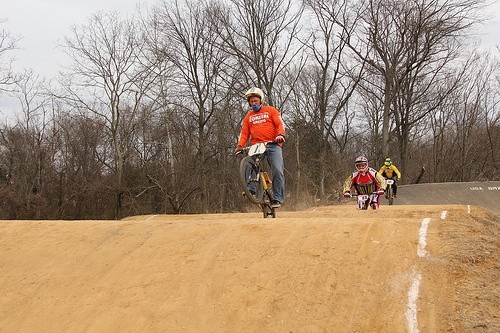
[352,192,377,210]
[383,176,395,205]
[234,141,275,218]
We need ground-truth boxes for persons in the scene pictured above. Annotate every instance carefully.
[235,87,287,208]
[342,156,401,211]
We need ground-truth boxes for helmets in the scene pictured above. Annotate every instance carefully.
[354,157,368,173]
[245,87,265,105]
[384,158,392,167]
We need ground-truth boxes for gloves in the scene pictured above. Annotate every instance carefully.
[377,190,385,195]
[234,147,243,155]
[275,135,286,143]
[344,193,350,199]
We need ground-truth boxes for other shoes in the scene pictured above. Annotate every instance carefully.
[272,202,281,207]
[242,190,255,197]
[394,195,397,198]
